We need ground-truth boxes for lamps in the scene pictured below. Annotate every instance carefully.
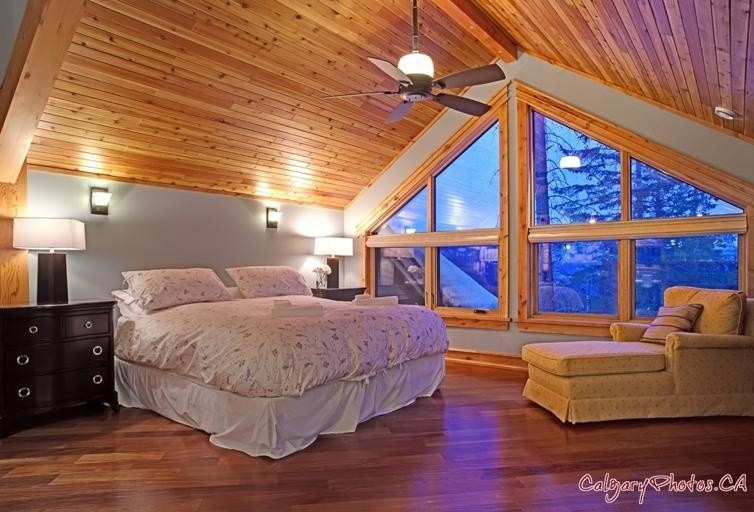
[90,187,112,214]
[314,237,354,288]
[397,53,434,79]
[13,217,86,304]
[266,207,277,228]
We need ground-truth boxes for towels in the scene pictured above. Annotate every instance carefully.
[270,304,323,318]
[352,294,398,306]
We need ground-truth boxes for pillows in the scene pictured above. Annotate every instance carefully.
[120,268,231,310]
[110,290,143,317]
[640,303,703,344]
[225,266,313,299]
[664,285,746,335]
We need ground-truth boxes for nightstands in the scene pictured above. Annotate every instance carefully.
[1,301,120,439]
[311,287,366,301]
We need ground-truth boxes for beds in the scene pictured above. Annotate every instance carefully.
[114,286,449,459]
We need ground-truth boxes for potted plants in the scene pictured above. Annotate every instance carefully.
[313,265,331,289]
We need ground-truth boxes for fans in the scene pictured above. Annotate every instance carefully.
[320,1,505,124]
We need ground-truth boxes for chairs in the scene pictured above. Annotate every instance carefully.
[522,298,754,425]
